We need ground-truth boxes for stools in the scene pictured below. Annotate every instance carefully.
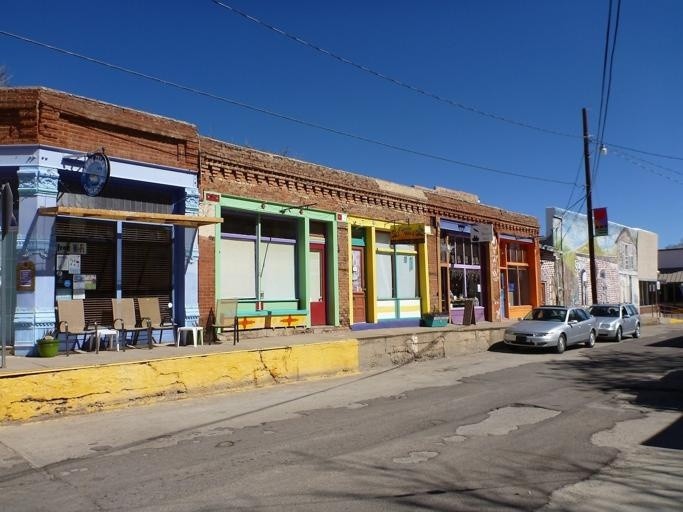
[175,326,204,348]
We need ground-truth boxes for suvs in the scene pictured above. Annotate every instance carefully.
[583,302,643,343]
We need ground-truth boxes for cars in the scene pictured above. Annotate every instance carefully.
[502,304,603,355]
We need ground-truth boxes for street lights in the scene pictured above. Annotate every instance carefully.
[580,107,608,305]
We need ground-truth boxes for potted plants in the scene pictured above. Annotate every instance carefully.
[35,331,58,357]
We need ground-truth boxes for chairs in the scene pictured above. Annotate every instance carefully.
[55,296,178,357]
[205,297,238,345]
